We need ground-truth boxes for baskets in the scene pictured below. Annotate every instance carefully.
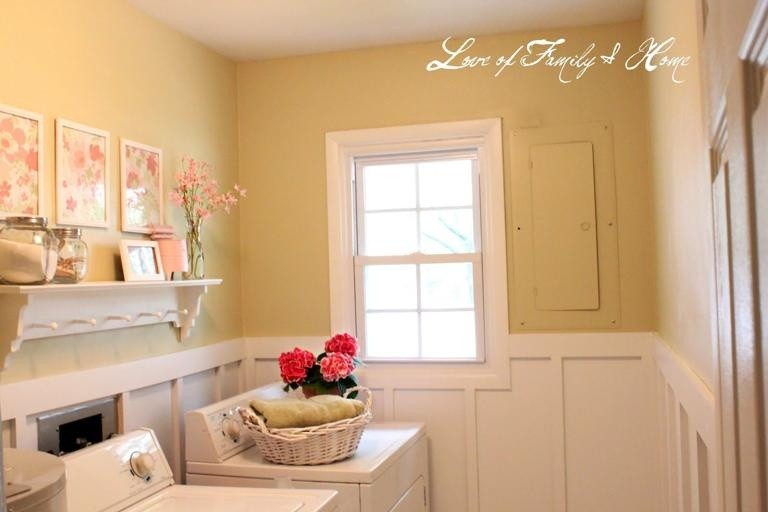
[238,387,373,466]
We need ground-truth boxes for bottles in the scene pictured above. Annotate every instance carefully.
[0,216,58,284]
[41,227,89,284]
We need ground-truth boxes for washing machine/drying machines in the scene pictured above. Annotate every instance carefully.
[184,383,430,512]
[59,427,341,511]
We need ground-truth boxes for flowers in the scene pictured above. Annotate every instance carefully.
[169,155,247,277]
[277,332,363,399]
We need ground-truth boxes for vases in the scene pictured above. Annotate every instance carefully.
[181,216,204,279]
[302,382,340,398]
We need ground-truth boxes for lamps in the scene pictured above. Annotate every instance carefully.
[159,239,189,281]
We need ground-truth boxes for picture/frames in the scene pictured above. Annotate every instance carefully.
[55,118,109,230]
[1,104,45,220]
[121,138,164,235]
[120,241,166,281]
